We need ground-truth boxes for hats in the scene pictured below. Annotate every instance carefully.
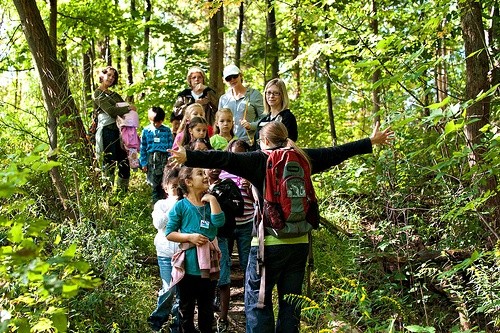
[223,64,241,78]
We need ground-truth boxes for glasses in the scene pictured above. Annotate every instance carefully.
[266,91,280,95]
[225,73,240,81]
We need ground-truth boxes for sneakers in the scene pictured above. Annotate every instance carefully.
[217,316,229,331]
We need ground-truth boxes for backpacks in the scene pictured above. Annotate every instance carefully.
[253,146,321,238]
[212,177,245,237]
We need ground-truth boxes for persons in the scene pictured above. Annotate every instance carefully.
[116,110,141,182]
[254,78,298,151]
[92,66,137,209]
[204,168,245,333]
[171,103,255,294]
[166,121,396,333]
[139,106,174,204]
[146,164,187,333]
[170,66,219,142]
[165,165,225,333]
[218,64,267,153]
